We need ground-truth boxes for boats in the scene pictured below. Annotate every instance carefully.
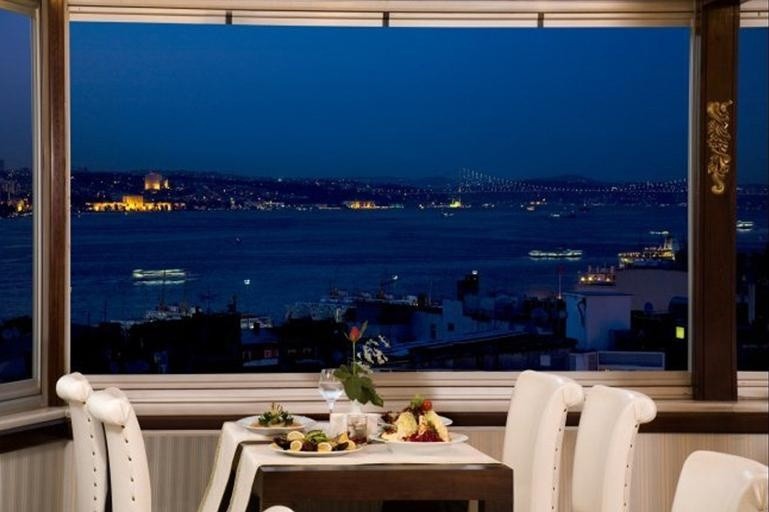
[735,220,755,230]
[526,248,583,258]
[131,266,187,282]
[579,229,676,287]
[120,286,417,334]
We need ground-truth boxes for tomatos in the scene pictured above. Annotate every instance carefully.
[421,399,432,411]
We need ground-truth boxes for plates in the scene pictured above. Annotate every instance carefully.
[270,439,364,457]
[377,413,456,427]
[238,415,319,433]
[383,432,470,446]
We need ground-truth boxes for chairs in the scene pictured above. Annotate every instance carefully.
[572,384,657,512]
[55,372,108,512]
[502,370,583,511]
[671,450,768,511]
[86,386,152,511]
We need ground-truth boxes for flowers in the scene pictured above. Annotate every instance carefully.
[343,320,369,370]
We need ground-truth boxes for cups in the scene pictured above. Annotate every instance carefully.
[329,413,345,434]
[347,414,369,441]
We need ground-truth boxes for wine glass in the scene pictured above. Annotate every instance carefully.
[319,368,349,419]
